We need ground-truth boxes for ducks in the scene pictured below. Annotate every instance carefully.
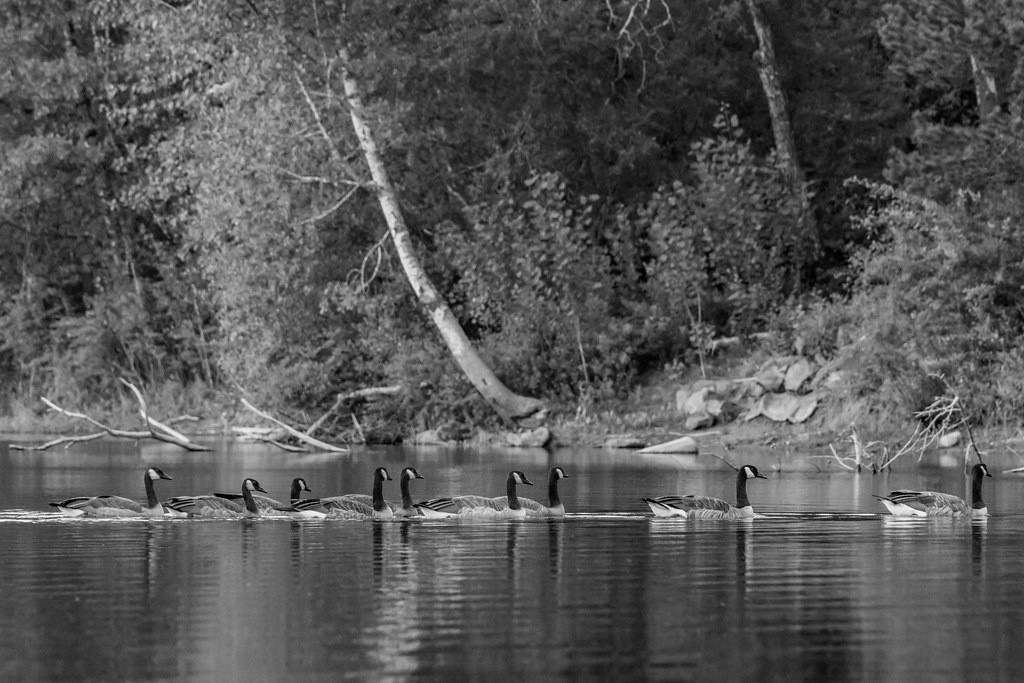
[870,462,994,519]
[639,464,769,520]
[45,465,569,522]
